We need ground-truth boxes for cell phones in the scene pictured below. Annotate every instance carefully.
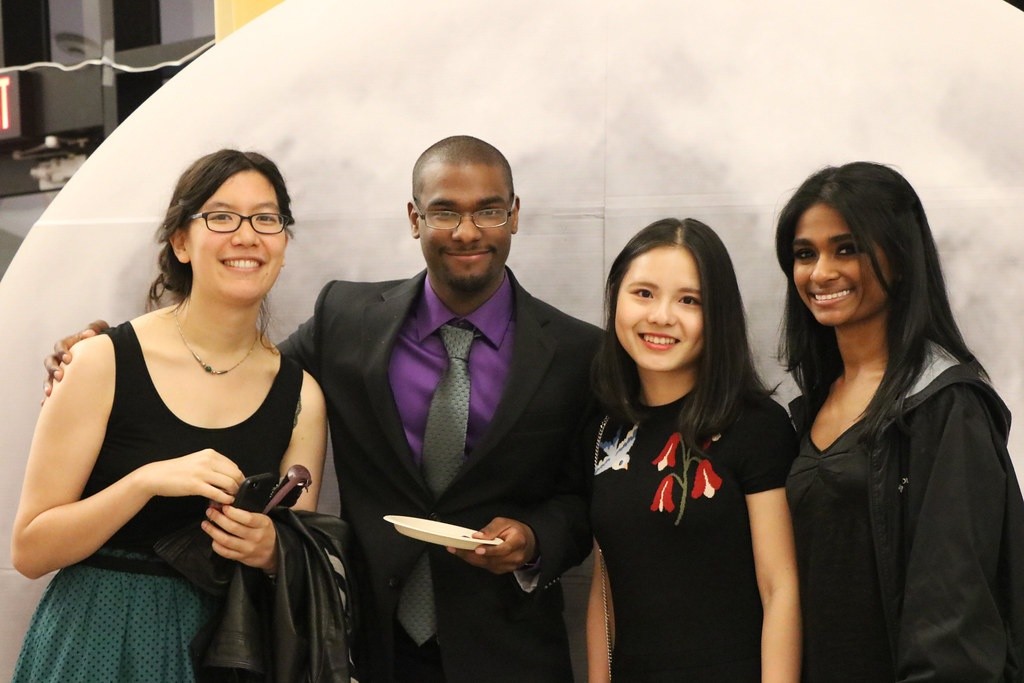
[232,473,277,514]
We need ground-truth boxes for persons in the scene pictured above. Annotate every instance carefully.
[775,163,1024,683]
[579,217,801,683]
[39,133,644,683]
[10,149,328,683]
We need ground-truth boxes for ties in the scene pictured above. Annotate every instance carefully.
[395,321,480,648]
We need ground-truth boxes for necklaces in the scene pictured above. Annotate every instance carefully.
[174,301,261,376]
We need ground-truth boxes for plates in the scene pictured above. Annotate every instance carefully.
[383,514,504,550]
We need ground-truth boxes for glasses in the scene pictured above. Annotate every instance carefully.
[413,198,515,229]
[184,210,290,233]
